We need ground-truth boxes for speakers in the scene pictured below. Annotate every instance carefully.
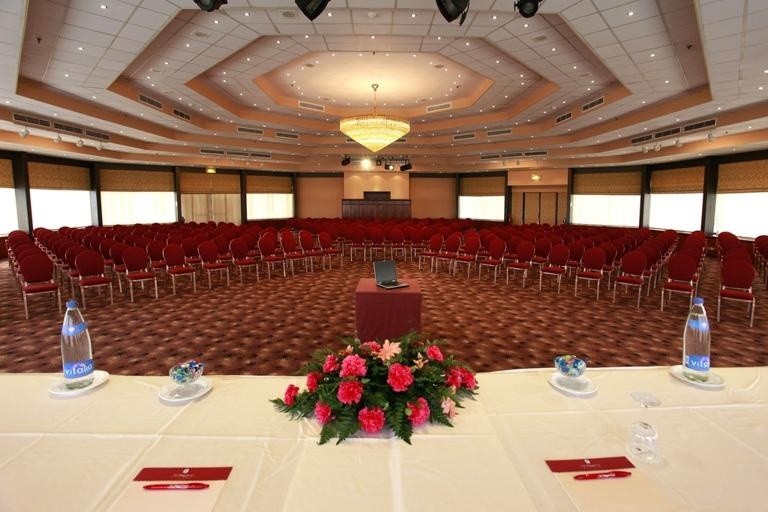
[400,163,412,171]
[342,158,351,166]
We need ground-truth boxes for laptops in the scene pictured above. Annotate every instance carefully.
[373,260,409,289]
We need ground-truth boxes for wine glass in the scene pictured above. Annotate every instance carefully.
[626,391,665,465]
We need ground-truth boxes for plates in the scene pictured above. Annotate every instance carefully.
[670,364,725,389]
[548,372,598,396]
[157,379,213,402]
[48,369,109,397]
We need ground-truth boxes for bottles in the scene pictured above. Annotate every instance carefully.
[682,296,711,381]
[61,300,94,388]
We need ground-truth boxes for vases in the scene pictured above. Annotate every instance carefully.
[347,419,397,439]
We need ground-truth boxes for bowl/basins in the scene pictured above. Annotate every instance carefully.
[169,363,203,390]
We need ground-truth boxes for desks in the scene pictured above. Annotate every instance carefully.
[0,366,768,512]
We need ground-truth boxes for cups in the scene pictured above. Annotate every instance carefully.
[554,356,586,386]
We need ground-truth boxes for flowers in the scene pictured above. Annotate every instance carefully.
[271,331,479,445]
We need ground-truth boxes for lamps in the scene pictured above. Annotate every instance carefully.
[193,0,227,12]
[436,0,471,27]
[531,173,541,181]
[675,139,682,148]
[18,125,29,138]
[54,133,61,144]
[206,168,217,174]
[339,84,411,153]
[706,130,716,144]
[294,0,331,22]
[95,142,104,151]
[653,145,661,152]
[640,146,648,154]
[341,154,411,172]
[75,137,83,148]
[514,0,543,18]
